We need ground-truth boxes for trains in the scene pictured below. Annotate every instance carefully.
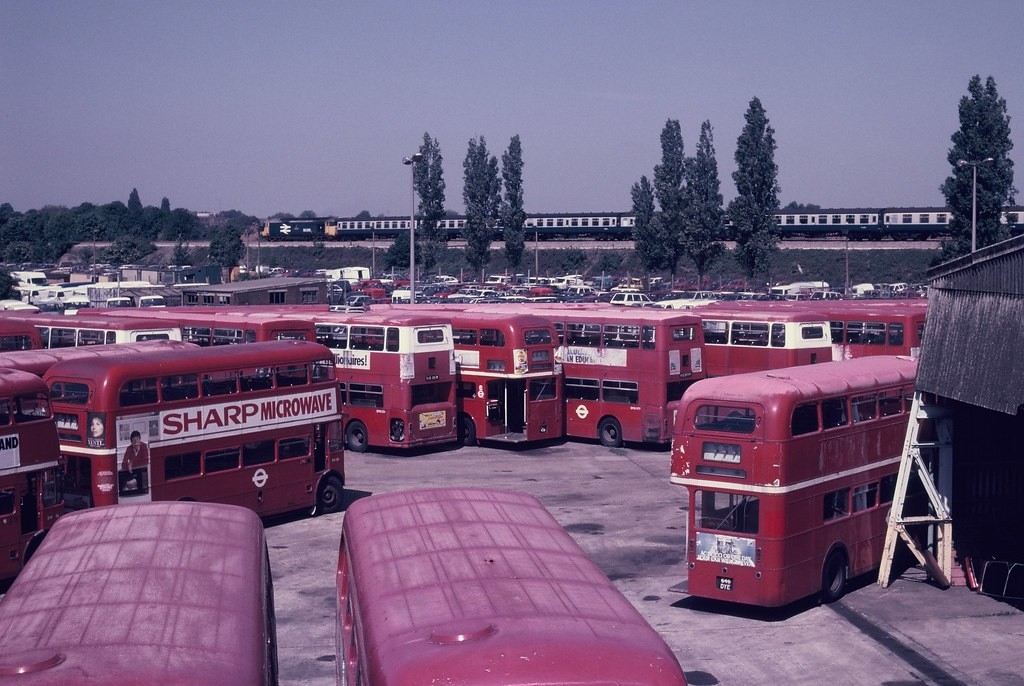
[267,211,1024,240]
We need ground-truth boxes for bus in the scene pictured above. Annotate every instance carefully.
[0,368,67,582]
[670,356,936,607]
[33,340,345,520]
[337,487,685,686]
[0,267,932,454]
[0,501,277,686]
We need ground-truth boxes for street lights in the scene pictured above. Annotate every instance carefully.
[957,157,995,252]
[402,153,424,303]
[257,225,265,280]
[92,229,100,283]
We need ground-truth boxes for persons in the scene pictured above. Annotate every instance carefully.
[122,431,148,473]
[88,416,105,448]
[402,355,413,378]
